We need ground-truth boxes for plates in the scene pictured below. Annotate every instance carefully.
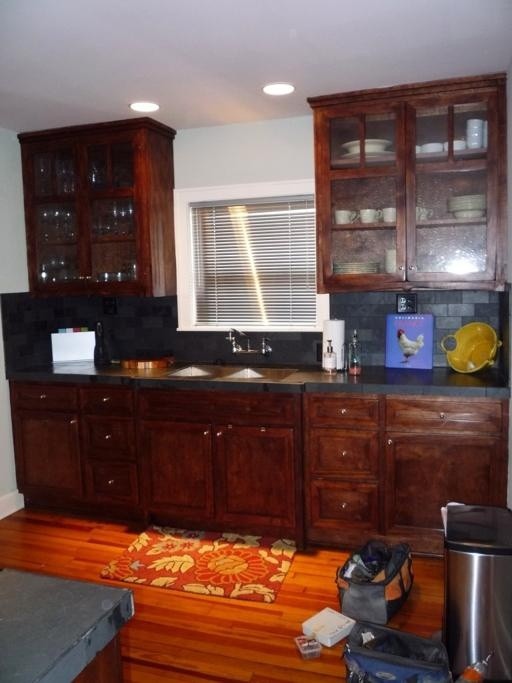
[334,261,380,273]
[339,151,396,160]
[447,195,487,217]
[341,138,392,153]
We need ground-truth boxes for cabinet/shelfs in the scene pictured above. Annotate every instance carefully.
[79,381,139,525]
[133,386,305,550]
[302,390,511,557]
[7,378,85,520]
[17,116,177,301]
[306,71,508,294]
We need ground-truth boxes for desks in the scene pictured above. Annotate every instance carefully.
[1,567,137,683]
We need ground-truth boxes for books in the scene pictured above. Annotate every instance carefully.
[301,606,357,648]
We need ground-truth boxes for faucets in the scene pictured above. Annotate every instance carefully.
[232,328,251,352]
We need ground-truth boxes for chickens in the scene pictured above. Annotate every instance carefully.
[397,329,424,363]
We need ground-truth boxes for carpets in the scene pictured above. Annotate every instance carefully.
[101,524,297,604]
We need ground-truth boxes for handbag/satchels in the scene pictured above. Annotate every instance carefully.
[335,539,414,626]
[342,622,452,682]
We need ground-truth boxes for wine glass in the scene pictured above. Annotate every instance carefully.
[39,251,78,282]
[91,198,135,234]
[37,203,77,244]
[33,151,78,197]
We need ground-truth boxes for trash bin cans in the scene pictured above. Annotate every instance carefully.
[443,504,512,683]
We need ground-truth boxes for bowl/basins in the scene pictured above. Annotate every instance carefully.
[466,118,483,149]
[420,142,444,152]
[385,250,396,273]
[443,140,466,151]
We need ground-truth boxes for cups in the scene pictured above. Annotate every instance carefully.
[98,259,136,282]
[334,210,356,224]
[360,209,382,223]
[383,208,396,222]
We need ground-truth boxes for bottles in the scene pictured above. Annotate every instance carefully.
[348,328,362,376]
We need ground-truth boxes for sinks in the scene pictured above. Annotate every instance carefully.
[160,364,221,379]
[222,367,299,383]
